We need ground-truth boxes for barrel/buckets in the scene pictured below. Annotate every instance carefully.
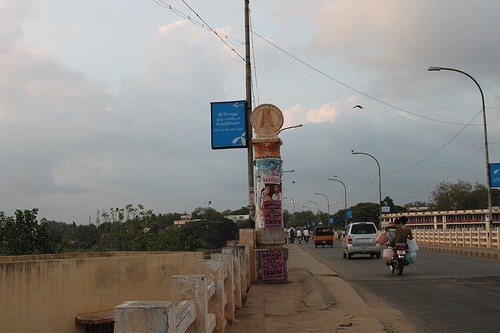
[376,230,395,260]
[407,238,419,251]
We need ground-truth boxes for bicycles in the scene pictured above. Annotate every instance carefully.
[289,232,310,244]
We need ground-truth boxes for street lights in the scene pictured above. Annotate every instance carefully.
[327,178,348,229]
[427,66,493,232]
[351,151,382,230]
[301,192,330,226]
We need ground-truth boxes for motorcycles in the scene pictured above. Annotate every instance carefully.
[312,226,335,248]
[384,230,414,276]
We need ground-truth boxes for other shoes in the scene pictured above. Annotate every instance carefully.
[405,260,409,265]
[386,261,392,265]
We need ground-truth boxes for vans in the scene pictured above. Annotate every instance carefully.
[341,222,381,260]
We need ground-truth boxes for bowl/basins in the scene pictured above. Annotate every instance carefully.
[405,253,413,265]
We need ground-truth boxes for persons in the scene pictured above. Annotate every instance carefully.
[337,228,341,239]
[297,229,302,244]
[290,227,295,244]
[384,216,413,266]
[303,229,310,243]
[264,184,271,201]
[269,161,280,175]
[250,191,254,204]
[260,188,264,208]
[272,185,281,200]
